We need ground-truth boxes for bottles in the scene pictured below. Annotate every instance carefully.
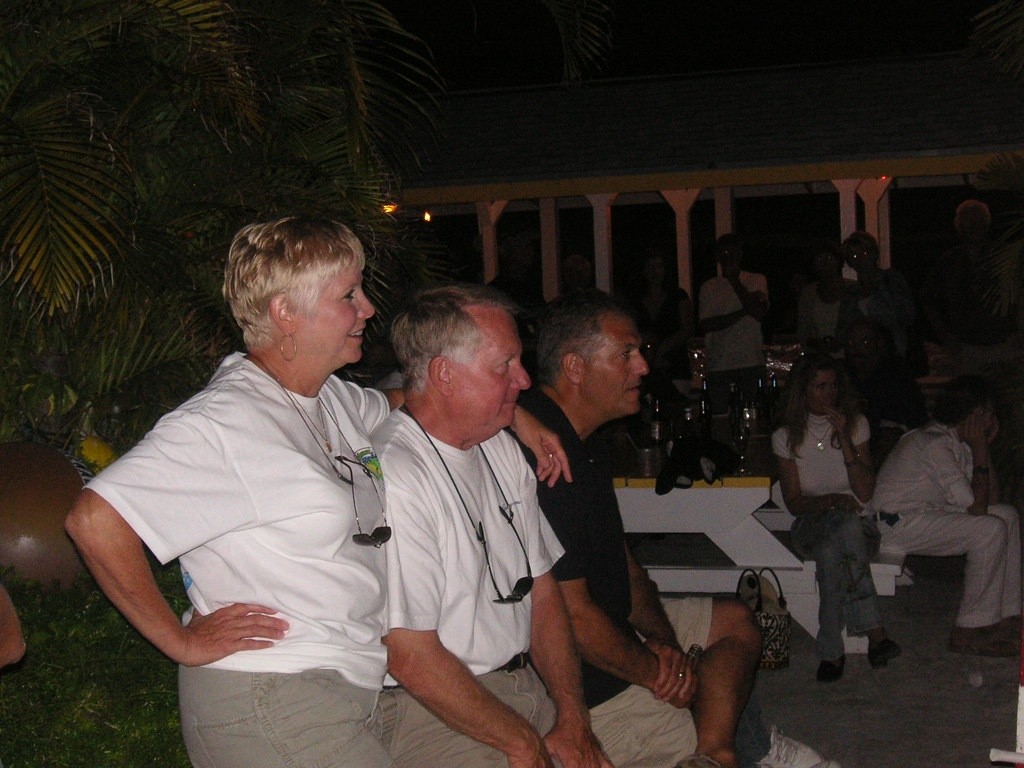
[698,400,712,439]
[651,400,663,444]
[699,380,711,407]
[682,404,692,437]
[770,379,785,425]
[756,379,768,424]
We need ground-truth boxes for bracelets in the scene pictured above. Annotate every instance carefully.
[822,496,824,509]
[845,456,862,466]
[973,466,988,473]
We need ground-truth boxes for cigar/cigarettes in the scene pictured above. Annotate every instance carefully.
[823,407,836,414]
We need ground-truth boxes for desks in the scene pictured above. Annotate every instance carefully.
[612,411,873,658]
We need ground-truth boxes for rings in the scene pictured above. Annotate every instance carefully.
[679,672,686,678]
[838,415,842,418]
[549,454,552,457]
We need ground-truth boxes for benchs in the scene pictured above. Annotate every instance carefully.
[871,551,913,596]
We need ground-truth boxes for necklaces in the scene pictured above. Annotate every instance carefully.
[275,375,332,453]
[806,425,832,451]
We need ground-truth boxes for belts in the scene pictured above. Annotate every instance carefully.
[872,512,890,521]
[495,653,527,671]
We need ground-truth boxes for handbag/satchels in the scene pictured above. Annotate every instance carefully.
[736,568,792,670]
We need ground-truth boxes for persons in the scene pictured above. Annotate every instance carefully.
[369,286,613,767]
[771,352,901,682]
[872,375,1024,657]
[65,214,572,768]
[504,292,839,768]
[565,200,1024,466]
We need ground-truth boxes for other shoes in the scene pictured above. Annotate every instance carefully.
[948,629,1021,658]
[996,617,1021,638]
[866,638,899,669]
[817,654,845,682]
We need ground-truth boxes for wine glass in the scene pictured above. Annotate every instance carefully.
[731,427,752,474]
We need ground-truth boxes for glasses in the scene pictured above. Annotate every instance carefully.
[337,455,392,548]
[852,251,869,258]
[479,506,533,604]
[817,256,832,261]
